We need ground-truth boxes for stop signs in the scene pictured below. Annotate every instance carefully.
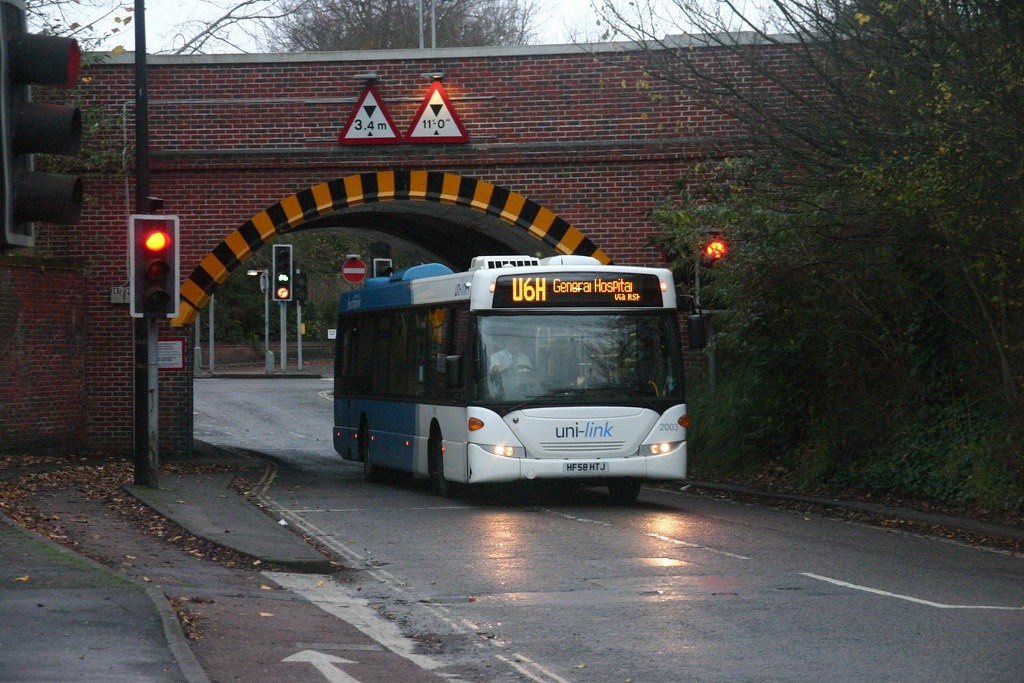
[342,259,367,283]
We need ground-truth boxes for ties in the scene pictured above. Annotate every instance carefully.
[511,356,516,365]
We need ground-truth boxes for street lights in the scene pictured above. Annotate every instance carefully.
[247,268,277,379]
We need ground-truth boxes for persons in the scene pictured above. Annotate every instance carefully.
[487,333,533,399]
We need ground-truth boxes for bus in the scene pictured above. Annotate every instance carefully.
[329,252,708,505]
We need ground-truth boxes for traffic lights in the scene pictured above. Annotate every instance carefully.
[295,274,308,300]
[273,245,292,302]
[132,215,178,318]
[701,233,729,262]
[1,0,84,251]
